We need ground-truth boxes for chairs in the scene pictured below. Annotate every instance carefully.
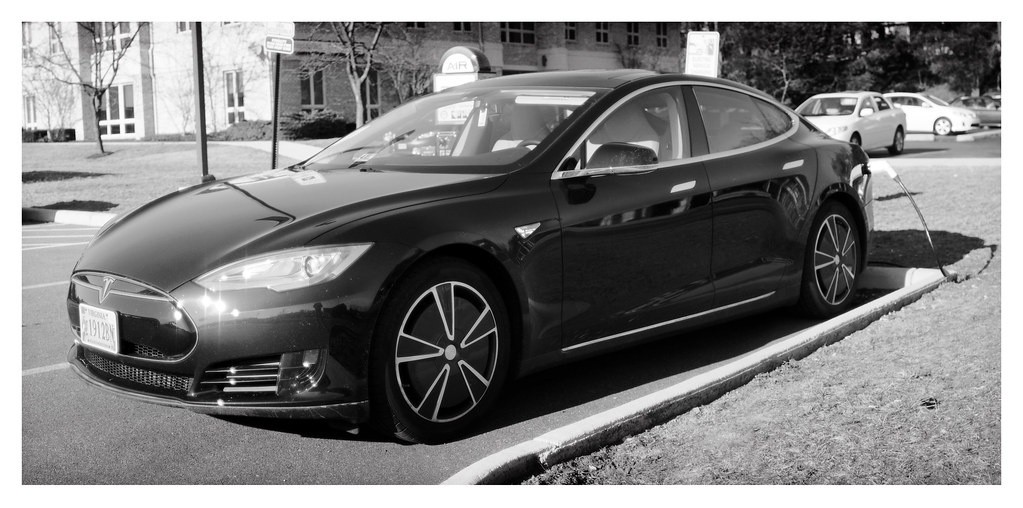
[824,102,841,114]
[575,101,658,170]
[491,103,551,150]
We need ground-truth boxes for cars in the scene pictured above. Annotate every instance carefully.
[63,63,875,450]
[792,89,907,156]
[949,94,1001,128]
[867,91,981,136]
